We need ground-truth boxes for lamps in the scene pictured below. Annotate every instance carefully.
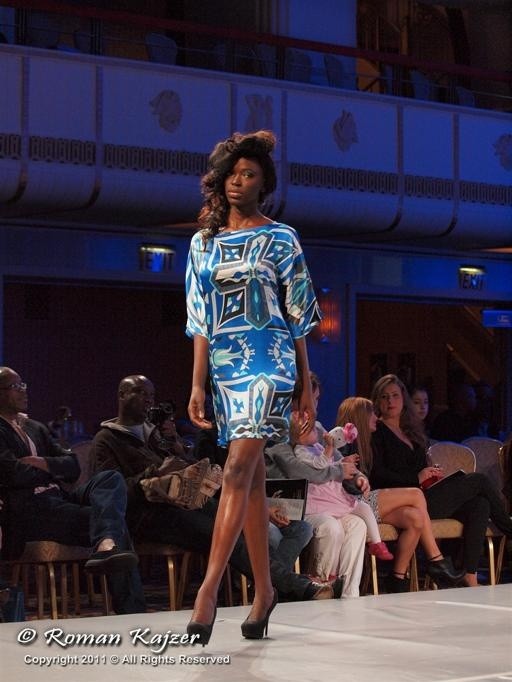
[311,286,342,348]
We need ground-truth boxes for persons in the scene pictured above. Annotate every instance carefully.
[336,397,468,593]
[288,409,394,584]
[0,365,147,615]
[328,423,358,448]
[369,374,512,586]
[184,129,323,648]
[89,373,347,599]
[405,382,442,451]
[262,369,371,599]
[258,440,314,604]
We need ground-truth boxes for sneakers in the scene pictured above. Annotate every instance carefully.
[85,545,137,577]
[368,542,394,561]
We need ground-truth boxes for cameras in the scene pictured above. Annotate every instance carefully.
[146,402,175,425]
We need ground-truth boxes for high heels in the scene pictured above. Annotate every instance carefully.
[384,571,410,594]
[424,558,467,589]
[240,587,277,639]
[187,606,218,648]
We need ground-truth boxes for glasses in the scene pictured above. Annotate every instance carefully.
[0,382,27,391]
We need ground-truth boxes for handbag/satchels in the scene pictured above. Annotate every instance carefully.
[138,457,223,511]
[0,589,24,622]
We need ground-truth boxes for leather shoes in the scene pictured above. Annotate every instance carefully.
[464,571,478,587]
[302,582,334,601]
[320,574,347,599]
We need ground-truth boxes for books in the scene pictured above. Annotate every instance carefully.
[425,469,468,491]
[264,477,309,521]
